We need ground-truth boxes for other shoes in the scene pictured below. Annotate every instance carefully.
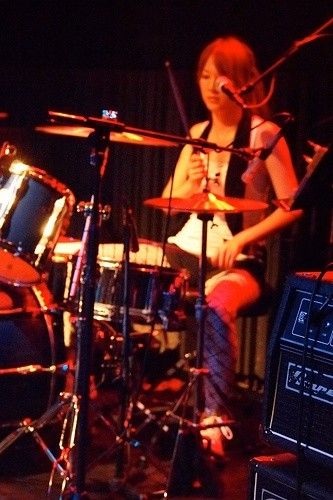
[200,414,233,457]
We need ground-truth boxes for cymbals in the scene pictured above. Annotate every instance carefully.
[35,125,179,148]
[143,193,269,213]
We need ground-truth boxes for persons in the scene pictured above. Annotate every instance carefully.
[160,36,305,460]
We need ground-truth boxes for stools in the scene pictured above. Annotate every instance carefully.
[181,283,277,417]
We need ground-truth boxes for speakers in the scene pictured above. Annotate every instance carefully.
[246,275,333,500]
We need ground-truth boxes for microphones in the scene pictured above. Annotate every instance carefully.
[214,76,245,110]
[122,199,140,253]
[240,118,295,184]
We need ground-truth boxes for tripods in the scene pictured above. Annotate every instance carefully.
[0,112,260,500]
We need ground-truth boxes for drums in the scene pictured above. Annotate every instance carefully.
[0,282,69,468]
[62,257,190,321]
[0,160,77,287]
